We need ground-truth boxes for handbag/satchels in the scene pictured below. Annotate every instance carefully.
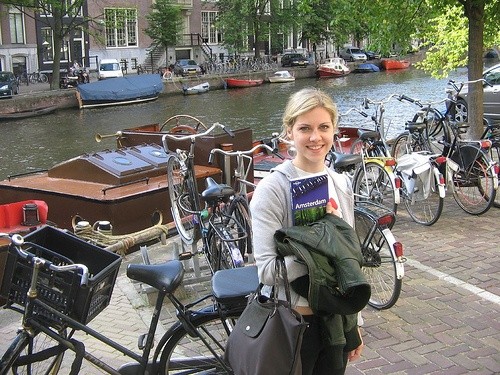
[395,152,456,202]
[225,259,308,375]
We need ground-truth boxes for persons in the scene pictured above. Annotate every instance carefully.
[312,43,316,52]
[194,54,201,66]
[167,55,181,75]
[246,88,363,375]
[72,60,80,69]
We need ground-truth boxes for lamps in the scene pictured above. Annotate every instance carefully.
[22,204,40,225]
[42,41,49,50]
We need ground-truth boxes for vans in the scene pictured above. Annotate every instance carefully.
[95,58,124,80]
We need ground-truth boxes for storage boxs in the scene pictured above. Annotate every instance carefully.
[0,224,122,330]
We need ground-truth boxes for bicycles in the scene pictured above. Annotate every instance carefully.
[208,142,285,262]
[16,71,49,86]
[202,54,278,75]
[161,121,248,278]
[0,223,260,374]
[270,79,500,311]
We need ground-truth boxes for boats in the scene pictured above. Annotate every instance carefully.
[314,57,351,79]
[380,58,412,71]
[74,72,166,109]
[267,70,296,83]
[224,77,264,89]
[181,81,210,97]
[354,62,381,73]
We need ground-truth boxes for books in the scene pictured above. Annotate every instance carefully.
[287,170,329,227]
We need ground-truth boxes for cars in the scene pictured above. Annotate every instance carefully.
[281,53,310,68]
[0,71,20,100]
[445,63,500,128]
[174,58,204,77]
[340,46,367,62]
[366,50,382,60]
